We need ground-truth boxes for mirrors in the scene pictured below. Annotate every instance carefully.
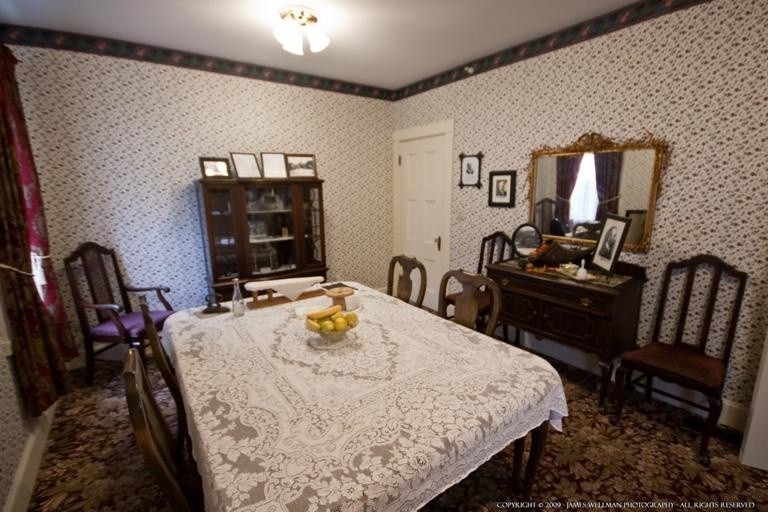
[528,132,668,255]
[512,223,543,257]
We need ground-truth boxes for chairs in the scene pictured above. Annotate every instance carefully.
[386,255,427,308]
[438,269,503,336]
[138,292,194,456]
[534,198,559,235]
[446,231,520,345]
[123,348,206,512]
[610,254,749,467]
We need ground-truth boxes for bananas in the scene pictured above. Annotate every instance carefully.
[305,305,343,319]
[306,318,321,332]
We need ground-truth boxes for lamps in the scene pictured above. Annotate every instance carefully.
[271,5,333,56]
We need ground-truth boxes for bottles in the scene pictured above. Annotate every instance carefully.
[233,278,244,316]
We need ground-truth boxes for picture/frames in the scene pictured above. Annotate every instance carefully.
[488,170,516,207]
[261,152,287,179]
[590,212,632,278]
[229,152,262,179]
[625,210,646,246]
[199,156,232,178]
[285,154,317,179]
[458,151,484,189]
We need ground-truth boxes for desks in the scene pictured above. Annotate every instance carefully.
[484,253,648,406]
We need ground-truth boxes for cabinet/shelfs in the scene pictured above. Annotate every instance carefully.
[195,178,329,303]
[64,242,179,386]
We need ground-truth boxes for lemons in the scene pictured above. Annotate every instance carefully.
[330,312,344,321]
[319,317,330,322]
[322,320,335,332]
[335,317,346,331]
[346,314,357,327]
